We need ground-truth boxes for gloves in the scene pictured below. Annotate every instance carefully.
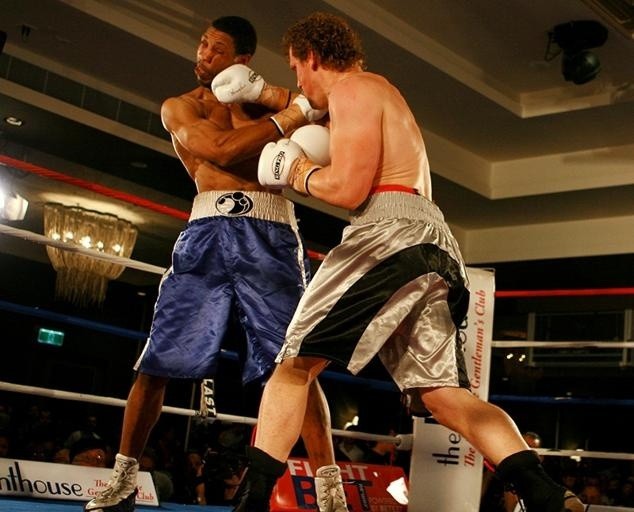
[257,138,323,198]
[210,63,291,112]
[290,124,332,168]
[269,93,329,138]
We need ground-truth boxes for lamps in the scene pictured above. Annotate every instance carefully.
[36,198,143,307]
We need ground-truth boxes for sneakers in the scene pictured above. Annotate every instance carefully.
[82,453,139,512]
[314,464,349,512]
[496,450,585,512]
[231,485,273,512]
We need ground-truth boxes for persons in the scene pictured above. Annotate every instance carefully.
[1,418,632,510]
[84,14,347,511]
[210,14,592,511]
